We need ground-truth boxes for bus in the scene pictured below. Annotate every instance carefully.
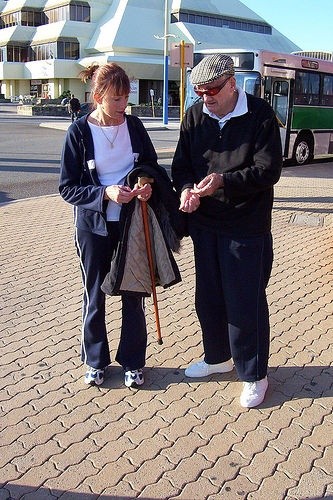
[182,48,333,165]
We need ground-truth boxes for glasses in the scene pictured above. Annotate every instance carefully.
[194,74,233,97]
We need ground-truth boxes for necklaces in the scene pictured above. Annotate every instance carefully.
[94,114,119,149]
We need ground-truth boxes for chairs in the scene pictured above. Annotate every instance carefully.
[15,93,36,103]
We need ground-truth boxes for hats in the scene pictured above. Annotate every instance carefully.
[190,54,235,85]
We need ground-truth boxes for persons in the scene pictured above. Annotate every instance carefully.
[57,62,170,388]
[171,53,283,409]
[68,94,81,124]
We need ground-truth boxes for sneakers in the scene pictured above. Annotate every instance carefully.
[123,367,144,387]
[240,375,268,407]
[84,365,108,386]
[184,358,233,377]
